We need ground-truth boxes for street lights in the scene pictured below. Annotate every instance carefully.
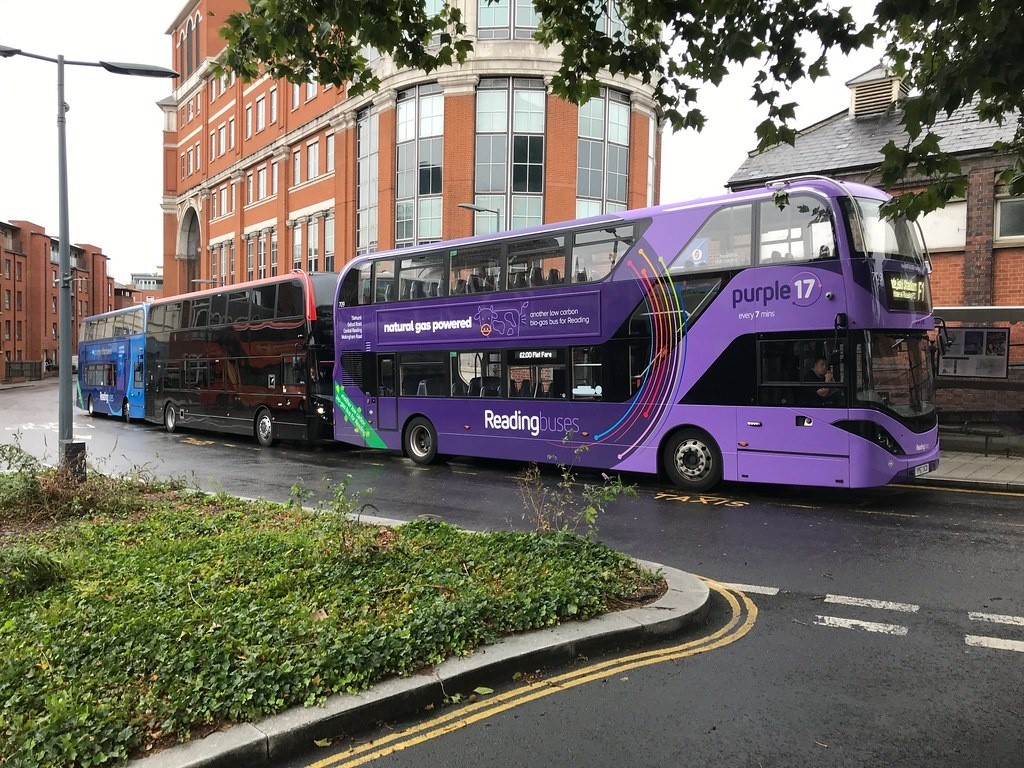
[1,44,180,441]
[457,204,500,233]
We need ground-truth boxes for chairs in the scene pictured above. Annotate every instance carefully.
[364,268,587,302]
[385,373,565,399]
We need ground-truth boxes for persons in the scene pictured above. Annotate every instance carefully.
[804,357,835,400]
[986,344,998,356]
[310,367,327,395]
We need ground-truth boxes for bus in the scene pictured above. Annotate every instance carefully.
[169,323,308,412]
[332,175,952,492]
[76,303,150,423]
[143,269,340,447]
